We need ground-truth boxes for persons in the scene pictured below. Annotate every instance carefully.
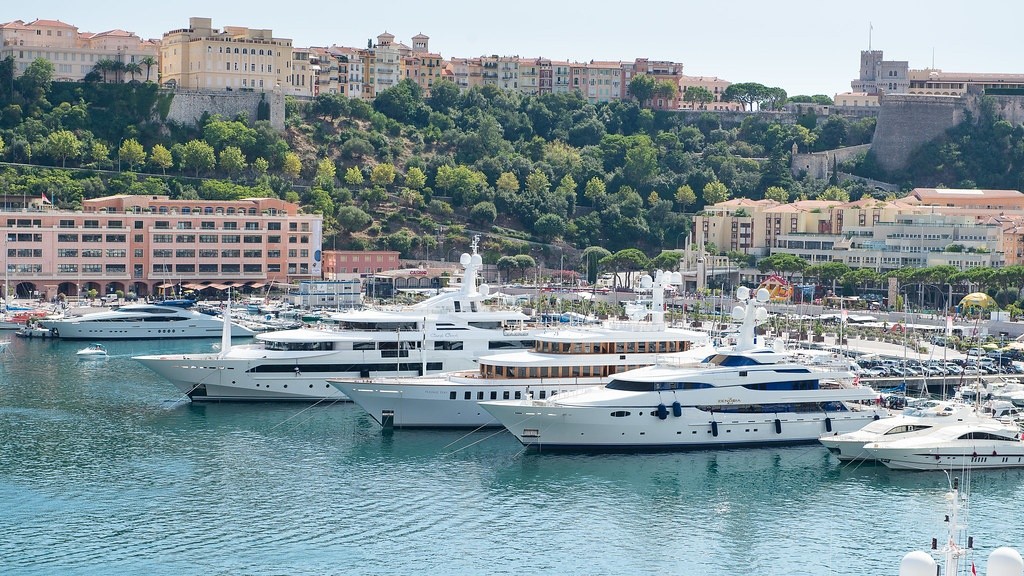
[95,346,101,350]
[861,395,883,407]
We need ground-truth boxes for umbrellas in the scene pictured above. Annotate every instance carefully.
[158,282,266,290]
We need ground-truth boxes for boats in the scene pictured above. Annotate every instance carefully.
[478,339,888,453]
[76,343,108,356]
[820,415,945,465]
[0,287,66,337]
[133,311,520,402]
[861,415,1023,472]
[33,299,252,340]
[325,324,713,429]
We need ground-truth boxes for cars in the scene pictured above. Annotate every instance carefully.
[533,289,1024,418]
